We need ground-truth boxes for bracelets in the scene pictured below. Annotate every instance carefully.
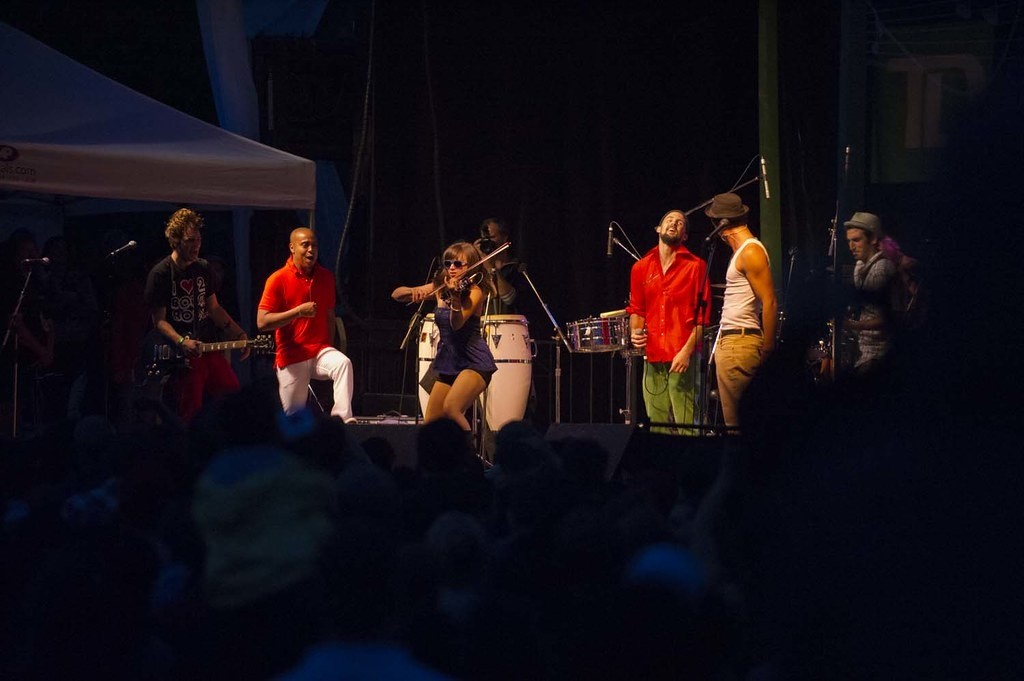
[178,338,184,345]
[237,332,247,340]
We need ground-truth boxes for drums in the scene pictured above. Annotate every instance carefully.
[478,314,532,432]
[417,312,468,423]
[565,316,631,354]
[619,332,646,357]
[758,311,791,348]
[804,341,854,363]
[702,323,721,358]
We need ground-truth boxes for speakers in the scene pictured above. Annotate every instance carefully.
[341,422,423,471]
[548,423,656,486]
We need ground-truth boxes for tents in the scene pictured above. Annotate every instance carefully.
[0,22,317,230]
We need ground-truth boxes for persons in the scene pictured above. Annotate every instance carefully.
[202,255,242,368]
[703,192,778,434]
[825,211,935,369]
[473,217,530,317]
[0,400,1023,681]
[143,207,251,420]
[629,209,713,437]
[256,227,354,426]
[391,240,497,434]
[0,227,55,404]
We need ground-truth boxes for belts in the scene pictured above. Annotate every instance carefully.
[722,329,761,336]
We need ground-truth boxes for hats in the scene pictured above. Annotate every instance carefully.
[844,212,883,240]
[704,193,750,218]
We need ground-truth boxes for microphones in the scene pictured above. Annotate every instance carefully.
[828,221,835,256]
[24,256,50,265]
[607,224,614,255]
[761,156,771,198]
[109,240,137,257]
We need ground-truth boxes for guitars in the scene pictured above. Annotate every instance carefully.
[828,252,853,382]
[140,329,274,376]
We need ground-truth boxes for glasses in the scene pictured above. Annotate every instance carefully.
[444,260,467,267]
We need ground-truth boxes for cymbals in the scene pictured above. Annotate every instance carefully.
[709,284,726,289]
[712,295,724,298]
